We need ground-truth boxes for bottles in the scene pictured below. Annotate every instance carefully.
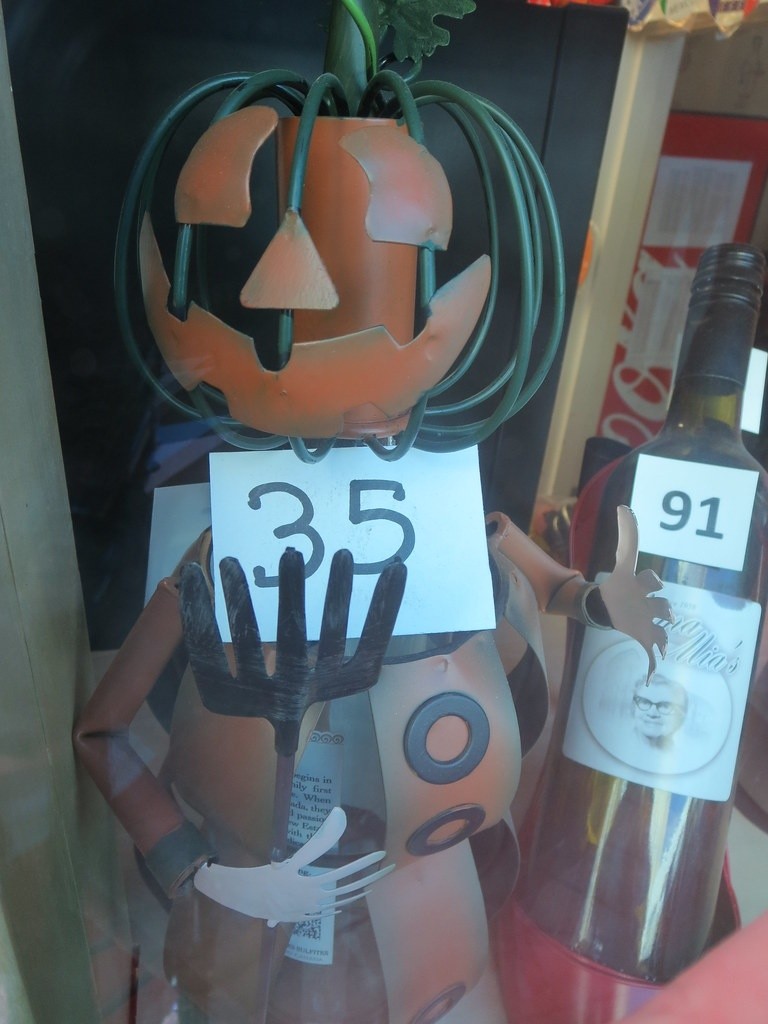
[521,243,768,984]
